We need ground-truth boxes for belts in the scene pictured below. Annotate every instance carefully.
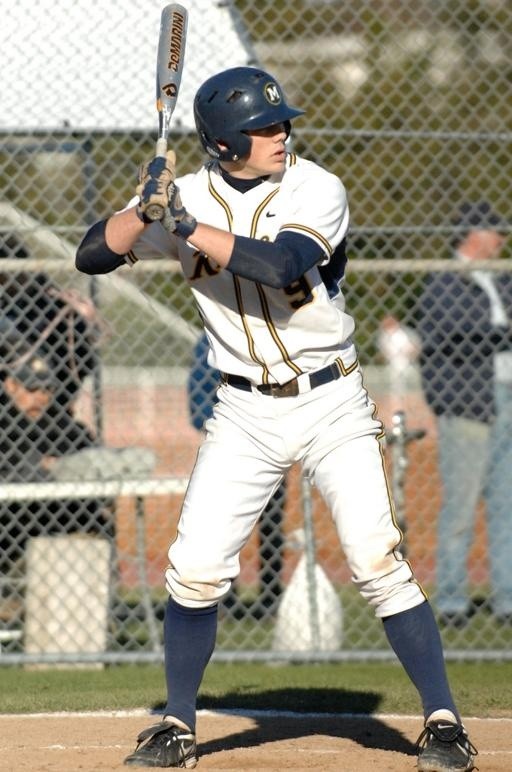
[224,361,340,401]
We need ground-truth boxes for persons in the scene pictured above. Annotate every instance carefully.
[74,64,482,772]
[0,227,96,398]
[0,348,116,647]
[379,200,512,629]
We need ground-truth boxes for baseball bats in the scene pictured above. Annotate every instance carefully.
[147,4,187,220]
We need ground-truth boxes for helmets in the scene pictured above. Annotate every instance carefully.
[192,66,306,162]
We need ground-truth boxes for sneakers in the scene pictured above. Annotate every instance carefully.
[414,717,477,771]
[123,720,198,769]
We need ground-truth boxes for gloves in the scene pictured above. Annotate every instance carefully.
[136,149,198,240]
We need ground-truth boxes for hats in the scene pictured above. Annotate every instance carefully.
[13,347,60,389]
